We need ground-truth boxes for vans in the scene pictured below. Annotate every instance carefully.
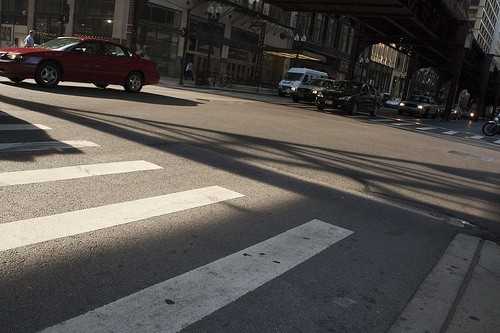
[278,67,329,96]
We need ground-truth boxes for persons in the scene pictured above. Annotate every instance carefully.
[185,61,194,81]
[24,30,36,47]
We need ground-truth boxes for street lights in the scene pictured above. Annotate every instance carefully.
[201,0,223,86]
[359,57,370,82]
[293,33,307,67]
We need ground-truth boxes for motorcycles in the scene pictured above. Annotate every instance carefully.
[482,113,500,136]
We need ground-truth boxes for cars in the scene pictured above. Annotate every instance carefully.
[0,35,161,93]
[315,80,381,115]
[399,95,439,118]
[450,104,461,120]
[290,78,338,105]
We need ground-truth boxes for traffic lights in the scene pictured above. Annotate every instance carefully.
[63,5,70,23]
